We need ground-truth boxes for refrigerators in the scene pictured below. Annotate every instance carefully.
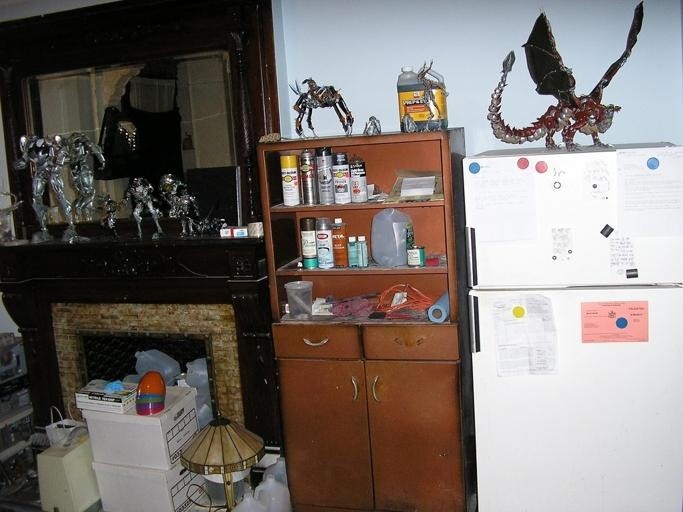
[460,141,682,512]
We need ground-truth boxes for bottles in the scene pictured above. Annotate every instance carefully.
[299,215,349,270]
[346,234,367,270]
[279,147,369,208]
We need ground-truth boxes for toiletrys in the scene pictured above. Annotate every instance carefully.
[357,236,368,268]
[347,236,358,268]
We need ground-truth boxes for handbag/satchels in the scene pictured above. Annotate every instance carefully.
[45,399,88,446]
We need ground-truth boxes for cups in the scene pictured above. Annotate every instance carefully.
[284,281,314,320]
[135,370,167,417]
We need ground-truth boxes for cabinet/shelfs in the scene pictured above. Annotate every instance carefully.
[271,324,467,510]
[0,366,37,512]
[257,130,459,324]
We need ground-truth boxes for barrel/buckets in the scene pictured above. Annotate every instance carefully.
[123,348,215,431]
[285,280,314,319]
[201,456,290,512]
[371,210,415,267]
[397,65,448,133]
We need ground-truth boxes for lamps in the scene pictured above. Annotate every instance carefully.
[180,410,266,511]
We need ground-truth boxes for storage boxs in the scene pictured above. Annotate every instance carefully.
[82,382,200,471]
[91,461,209,512]
[37,432,100,512]
